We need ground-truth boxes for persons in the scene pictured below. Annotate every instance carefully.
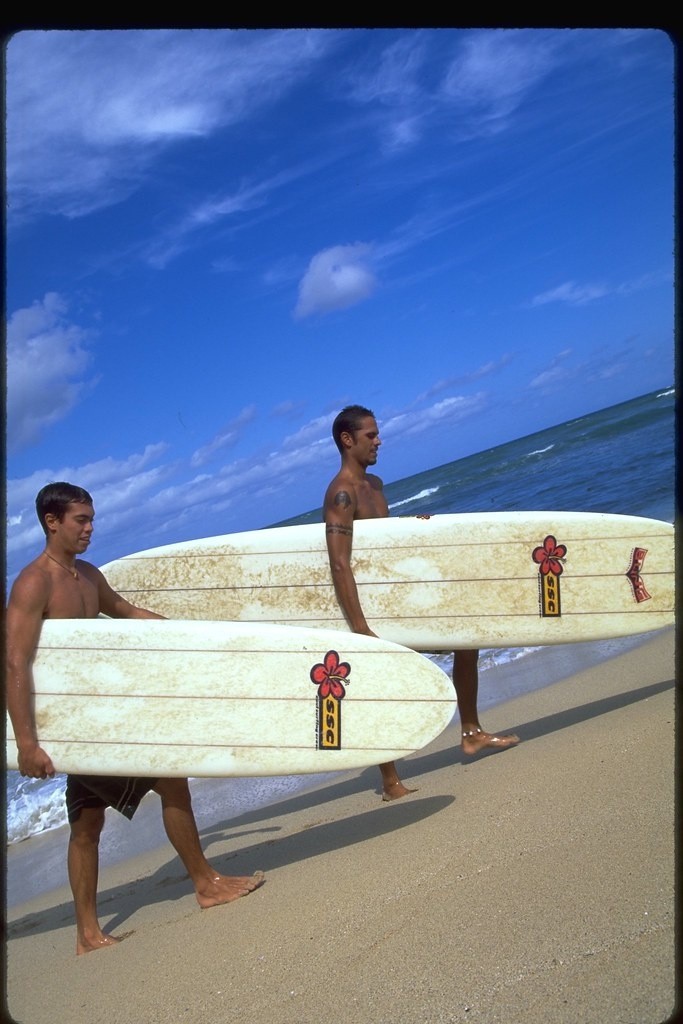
[2,483,265,954]
[322,406,519,797]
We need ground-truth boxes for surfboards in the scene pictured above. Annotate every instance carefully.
[97,511,676,651]
[0,619,460,778]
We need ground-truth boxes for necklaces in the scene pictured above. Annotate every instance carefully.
[43,548,80,579]
